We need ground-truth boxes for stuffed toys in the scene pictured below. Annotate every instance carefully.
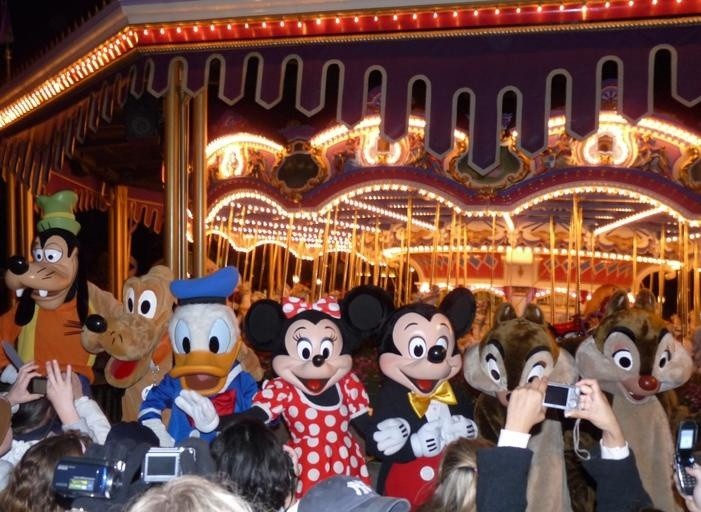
[0,188,701,512]
[1,190,701,512]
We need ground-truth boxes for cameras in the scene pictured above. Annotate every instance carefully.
[541,383,580,412]
[25,377,48,397]
[140,446,195,482]
[51,457,125,501]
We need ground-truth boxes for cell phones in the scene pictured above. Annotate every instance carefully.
[675,420,697,497]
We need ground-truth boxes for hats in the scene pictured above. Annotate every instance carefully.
[297,477,412,511]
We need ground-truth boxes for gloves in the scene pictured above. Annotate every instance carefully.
[69,439,163,511]
[180,436,217,480]
